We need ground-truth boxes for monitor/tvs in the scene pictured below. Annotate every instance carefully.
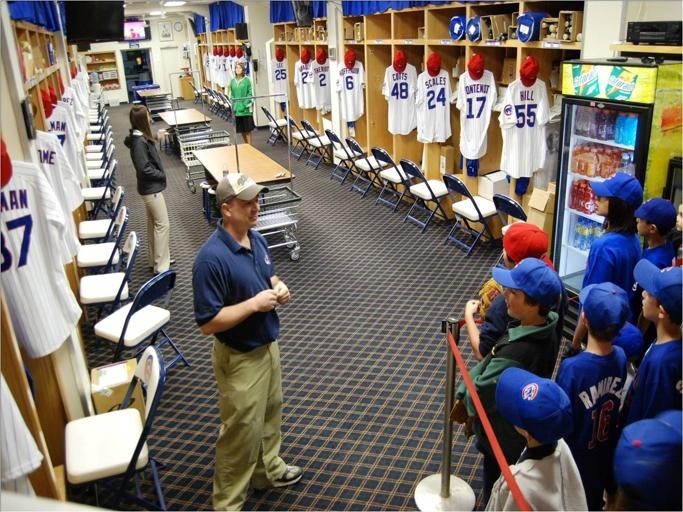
[123,20,151,42]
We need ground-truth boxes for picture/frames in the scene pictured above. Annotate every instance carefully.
[157,21,174,42]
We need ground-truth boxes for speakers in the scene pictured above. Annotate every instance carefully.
[236,23,248,40]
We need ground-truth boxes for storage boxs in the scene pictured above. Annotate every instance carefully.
[438,145,514,240]
[88,357,148,429]
[479,11,518,41]
[527,181,558,258]
[538,10,582,43]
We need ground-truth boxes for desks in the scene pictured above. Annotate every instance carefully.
[192,144,296,185]
[157,108,212,159]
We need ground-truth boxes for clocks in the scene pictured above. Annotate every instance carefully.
[174,22,183,32]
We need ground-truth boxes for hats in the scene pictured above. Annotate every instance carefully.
[216,171,269,202]
[427,53,441,76]
[301,48,311,64]
[275,48,285,62]
[495,367,572,441]
[394,48,406,71]
[345,50,356,68]
[467,55,484,79]
[40,79,64,118]
[520,57,539,86]
[492,222,561,303]
[615,411,682,490]
[317,47,326,64]
[213,46,244,58]
[578,173,683,331]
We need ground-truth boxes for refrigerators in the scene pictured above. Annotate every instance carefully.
[552,60,682,348]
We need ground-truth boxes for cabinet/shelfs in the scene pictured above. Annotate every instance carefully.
[84,51,121,91]
[196,28,248,93]
[11,21,89,324]
[272,1,583,242]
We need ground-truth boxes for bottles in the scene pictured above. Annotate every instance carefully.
[571,103,637,257]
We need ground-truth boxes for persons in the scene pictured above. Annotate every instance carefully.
[484,367,588,511]
[465,221,561,507]
[192,173,304,510]
[634,197,677,368]
[124,104,176,278]
[555,259,683,510]
[562,173,644,360]
[229,62,255,145]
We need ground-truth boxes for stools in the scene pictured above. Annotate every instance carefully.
[200,181,215,213]
[207,184,221,226]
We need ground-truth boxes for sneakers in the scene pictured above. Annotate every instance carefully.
[255,465,301,487]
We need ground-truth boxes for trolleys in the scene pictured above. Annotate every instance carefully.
[170,122,232,195]
[251,184,304,261]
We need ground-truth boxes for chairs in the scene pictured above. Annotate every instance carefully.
[283,113,320,163]
[77,184,126,244]
[371,145,424,213]
[81,158,119,221]
[94,268,189,392]
[261,106,292,146]
[76,205,130,276]
[345,134,388,199]
[441,172,499,260]
[80,229,142,331]
[64,344,165,511]
[325,129,367,185]
[188,80,232,122]
[82,90,116,169]
[400,158,453,235]
[301,119,331,170]
[490,192,528,269]
